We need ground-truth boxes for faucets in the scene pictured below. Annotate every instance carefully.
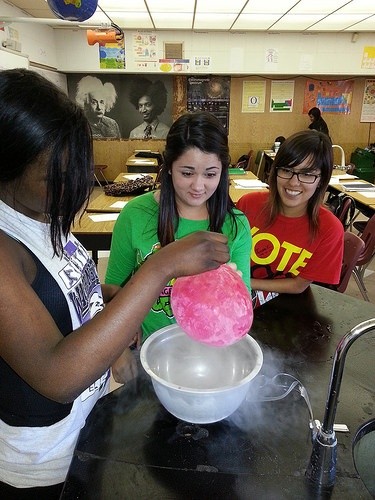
[304,318,375,488]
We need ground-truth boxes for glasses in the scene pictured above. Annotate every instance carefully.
[275,167,321,183]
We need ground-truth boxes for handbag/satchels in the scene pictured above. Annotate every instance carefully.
[104,175,154,197]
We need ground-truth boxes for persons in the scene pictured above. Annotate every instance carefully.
[76,76,121,136]
[0,68,230,498]
[309,107,333,144]
[236,130,344,310]
[130,77,170,140]
[105,111,252,384]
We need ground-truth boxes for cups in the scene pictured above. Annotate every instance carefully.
[273,141,280,153]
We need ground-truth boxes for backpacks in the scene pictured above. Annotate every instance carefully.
[324,191,356,232]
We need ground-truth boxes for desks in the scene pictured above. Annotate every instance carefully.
[228,171,268,205]
[328,169,375,217]
[70,151,160,265]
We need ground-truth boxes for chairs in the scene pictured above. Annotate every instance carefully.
[351,214,375,302]
[336,231,365,292]
[338,197,352,222]
[244,150,254,174]
[91,165,109,187]
[254,150,265,176]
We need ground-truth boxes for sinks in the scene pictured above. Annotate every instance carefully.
[351,417,375,498]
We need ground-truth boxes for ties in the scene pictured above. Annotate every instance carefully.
[144,125,152,137]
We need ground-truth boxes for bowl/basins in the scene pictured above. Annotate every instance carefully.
[139,322,264,426]
